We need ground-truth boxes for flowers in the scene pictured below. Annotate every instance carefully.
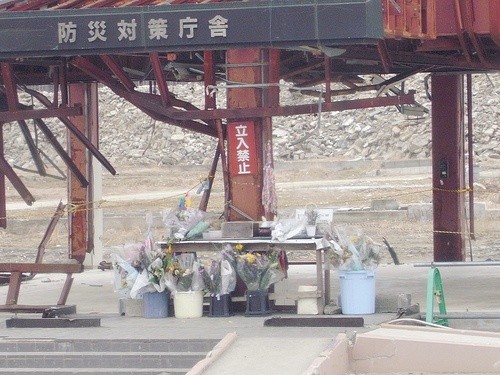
[110,193,383,300]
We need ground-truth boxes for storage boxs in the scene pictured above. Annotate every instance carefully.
[294,286,318,314]
[221,205,259,239]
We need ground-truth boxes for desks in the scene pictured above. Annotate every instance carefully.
[154,237,331,315]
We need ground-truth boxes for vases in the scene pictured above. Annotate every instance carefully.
[126,267,377,319]
